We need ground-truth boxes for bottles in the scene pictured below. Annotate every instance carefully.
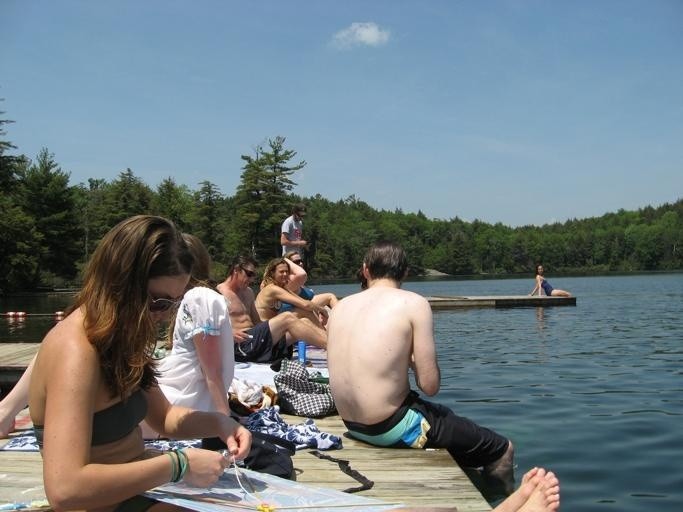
[298,340,306,367]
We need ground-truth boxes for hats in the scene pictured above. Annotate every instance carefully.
[295,204,307,215]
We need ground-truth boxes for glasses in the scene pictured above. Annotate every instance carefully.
[293,259,304,265]
[240,266,255,278]
[146,293,183,313]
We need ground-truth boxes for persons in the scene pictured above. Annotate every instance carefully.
[528,265,572,297]
